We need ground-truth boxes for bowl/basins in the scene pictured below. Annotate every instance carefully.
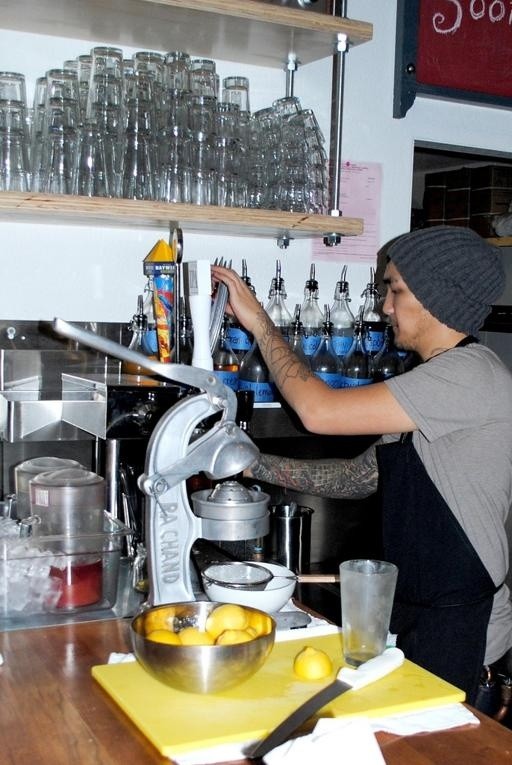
[129,601,277,695]
[201,559,298,613]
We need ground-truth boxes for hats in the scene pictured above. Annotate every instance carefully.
[385,224,507,336]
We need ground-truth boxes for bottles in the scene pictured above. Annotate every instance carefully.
[122,291,161,376]
[212,276,404,403]
[9,451,110,613]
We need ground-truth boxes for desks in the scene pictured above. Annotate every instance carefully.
[0,601,511,764]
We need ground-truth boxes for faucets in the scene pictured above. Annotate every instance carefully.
[0,494,17,518]
[18,515,41,541]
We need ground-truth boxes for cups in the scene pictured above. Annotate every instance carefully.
[338,558,399,666]
[270,499,316,576]
[0,44,330,215]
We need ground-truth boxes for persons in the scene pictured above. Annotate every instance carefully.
[209,223,512,726]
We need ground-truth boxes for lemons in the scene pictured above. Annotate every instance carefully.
[293,647,331,681]
[146,604,271,647]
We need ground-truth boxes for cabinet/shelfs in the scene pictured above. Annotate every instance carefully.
[3,0,372,250]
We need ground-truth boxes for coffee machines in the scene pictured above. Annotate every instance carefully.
[49,313,313,629]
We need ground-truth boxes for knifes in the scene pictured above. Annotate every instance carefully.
[249,645,405,759]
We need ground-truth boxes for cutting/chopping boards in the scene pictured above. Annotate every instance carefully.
[90,628,469,757]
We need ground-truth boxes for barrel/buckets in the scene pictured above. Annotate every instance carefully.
[29,468,108,610]
[14,455,83,522]
